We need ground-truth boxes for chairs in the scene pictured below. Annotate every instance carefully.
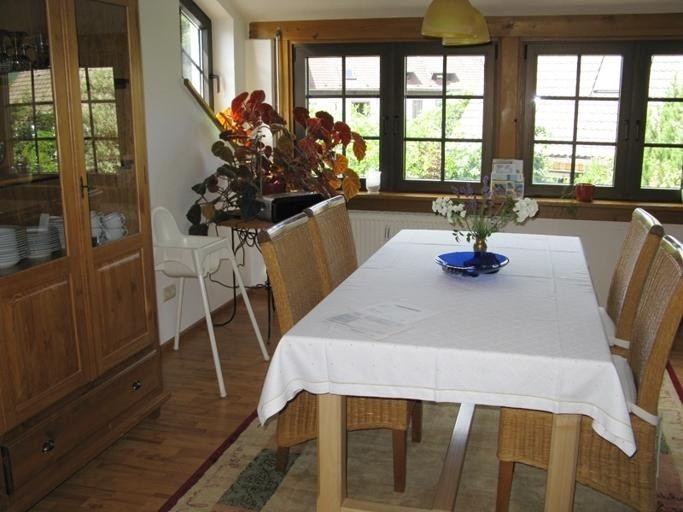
[305,194,358,297]
[257,212,423,493]
[151,206,270,399]
[598,208,664,358]
[496,234,683,512]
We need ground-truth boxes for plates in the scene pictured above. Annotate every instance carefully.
[0,216,66,269]
[434,251,509,277]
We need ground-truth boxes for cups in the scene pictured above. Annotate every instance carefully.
[365,170,382,192]
[89,210,128,249]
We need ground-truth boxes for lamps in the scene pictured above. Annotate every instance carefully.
[421,0,491,46]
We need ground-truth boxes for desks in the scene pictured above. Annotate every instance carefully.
[212,210,277,346]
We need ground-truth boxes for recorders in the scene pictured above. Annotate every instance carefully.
[261,191,325,223]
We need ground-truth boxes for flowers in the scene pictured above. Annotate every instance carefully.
[432,175,539,242]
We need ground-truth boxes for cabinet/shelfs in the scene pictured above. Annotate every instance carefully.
[0,0,172,512]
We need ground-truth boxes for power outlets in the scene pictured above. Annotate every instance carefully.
[163,285,176,302]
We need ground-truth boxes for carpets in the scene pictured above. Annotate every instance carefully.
[159,358,683,512]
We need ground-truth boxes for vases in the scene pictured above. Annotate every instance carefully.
[473,237,487,252]
[576,182,596,203]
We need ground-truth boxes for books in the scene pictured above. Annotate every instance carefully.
[489,158,525,201]
